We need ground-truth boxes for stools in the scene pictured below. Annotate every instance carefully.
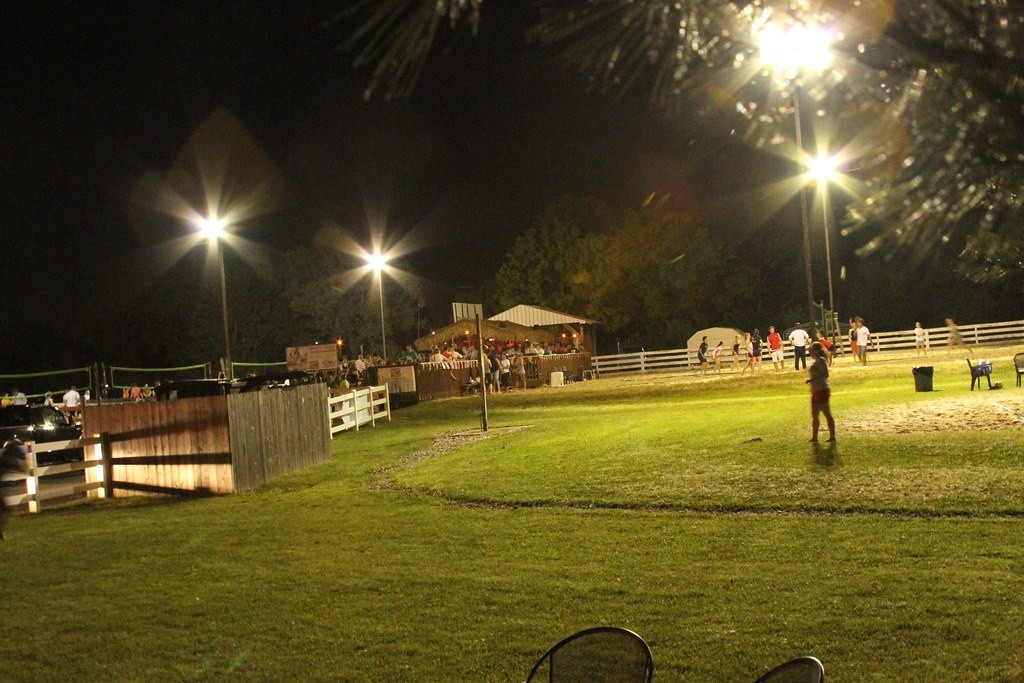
[582,369,596,381]
[461,383,475,396]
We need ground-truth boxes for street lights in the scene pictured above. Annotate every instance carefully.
[339,341,342,360]
[778,40,817,338]
[816,161,836,354]
[371,257,387,362]
[210,222,232,382]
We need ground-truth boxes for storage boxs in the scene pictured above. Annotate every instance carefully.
[975,363,992,375]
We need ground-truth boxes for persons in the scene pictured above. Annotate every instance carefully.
[698,335,710,376]
[123,378,173,399]
[63,386,81,417]
[945,318,972,355]
[806,341,836,442]
[816,332,834,366]
[767,326,785,372]
[44,391,53,406]
[741,328,764,377]
[914,321,925,355]
[849,316,875,365]
[732,336,740,372]
[322,340,585,423]
[11,387,27,405]
[713,341,727,375]
[788,322,809,370]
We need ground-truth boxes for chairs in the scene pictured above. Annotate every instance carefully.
[1013,353,1024,386]
[563,371,576,384]
[526,627,655,683]
[967,358,991,390]
[754,656,824,683]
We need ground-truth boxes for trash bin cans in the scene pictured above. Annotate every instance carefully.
[911,367,935,391]
